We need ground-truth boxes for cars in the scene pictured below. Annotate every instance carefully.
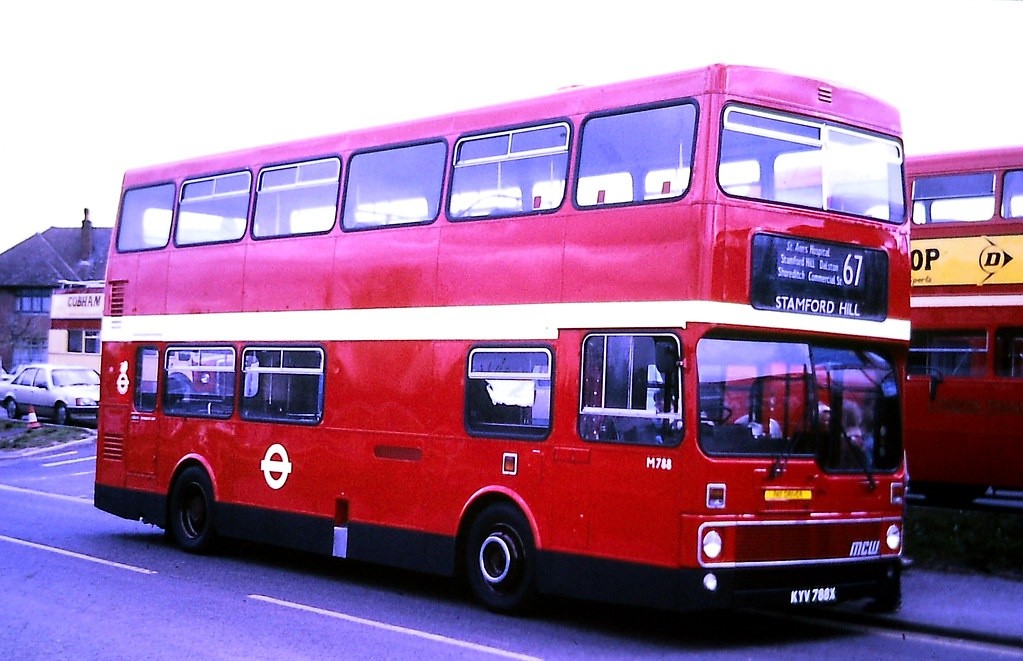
[0,365,102,425]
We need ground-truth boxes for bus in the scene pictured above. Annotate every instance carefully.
[720,144,1023,512]
[94,64,912,630]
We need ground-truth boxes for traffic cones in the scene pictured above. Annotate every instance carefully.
[25,404,42,430]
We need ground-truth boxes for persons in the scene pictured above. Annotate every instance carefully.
[810,400,875,468]
